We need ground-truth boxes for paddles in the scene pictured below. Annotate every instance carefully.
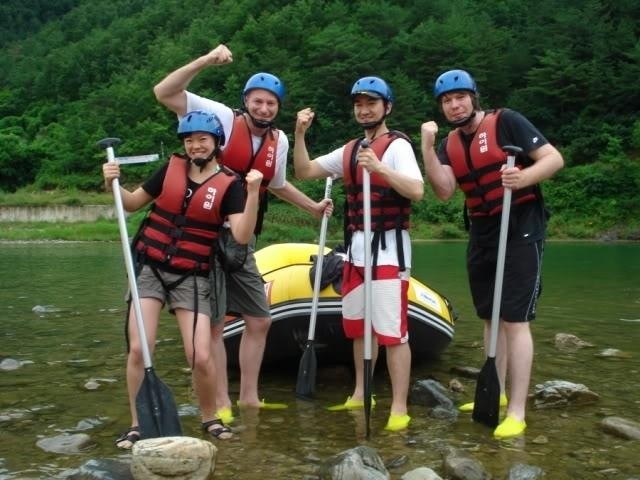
[362,139,371,441]
[297,173,337,399]
[471,145,523,428]
[98,137,183,439]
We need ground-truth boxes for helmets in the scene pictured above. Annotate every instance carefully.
[177,111,225,146]
[350,76,394,103]
[243,72,286,107]
[433,69,476,100]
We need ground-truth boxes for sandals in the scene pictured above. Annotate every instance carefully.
[201,418,231,441]
[115,427,141,451]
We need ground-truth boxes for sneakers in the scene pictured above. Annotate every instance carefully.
[328,395,376,411]
[493,417,527,437]
[459,394,508,411]
[237,398,288,409]
[384,414,411,430]
[216,409,233,424]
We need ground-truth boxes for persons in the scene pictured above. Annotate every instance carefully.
[292,74,425,432]
[151,42,336,425]
[99,108,264,453]
[419,69,566,441]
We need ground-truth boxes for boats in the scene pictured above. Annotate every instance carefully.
[224,242,454,366]
